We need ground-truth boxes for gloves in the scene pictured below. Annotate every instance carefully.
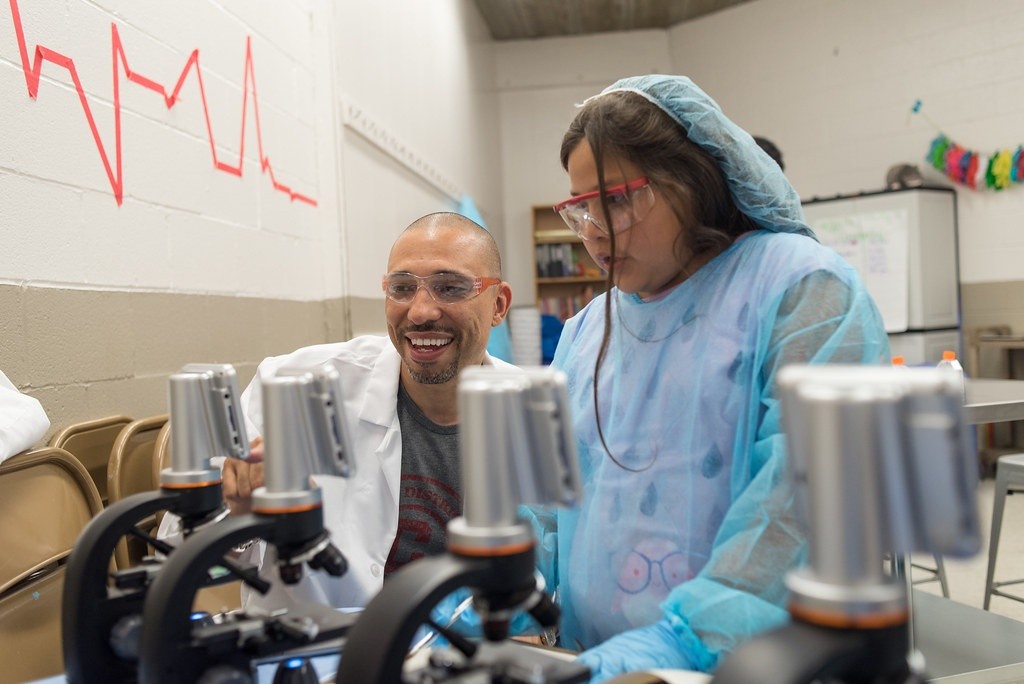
[581,610,716,684]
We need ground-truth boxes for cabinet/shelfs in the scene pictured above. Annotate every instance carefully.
[530,204,608,308]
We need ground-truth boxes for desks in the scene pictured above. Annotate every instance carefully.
[911,378,1024,599]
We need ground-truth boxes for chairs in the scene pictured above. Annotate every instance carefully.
[0,414,171,684]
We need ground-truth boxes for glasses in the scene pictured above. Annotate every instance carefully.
[553,178,657,240]
[379,271,503,305]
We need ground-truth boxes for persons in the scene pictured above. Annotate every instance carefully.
[423,73,891,684]
[155,212,559,649]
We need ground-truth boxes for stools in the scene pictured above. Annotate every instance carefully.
[982,454,1024,611]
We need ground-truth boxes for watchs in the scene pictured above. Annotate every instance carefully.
[539,627,557,648]
